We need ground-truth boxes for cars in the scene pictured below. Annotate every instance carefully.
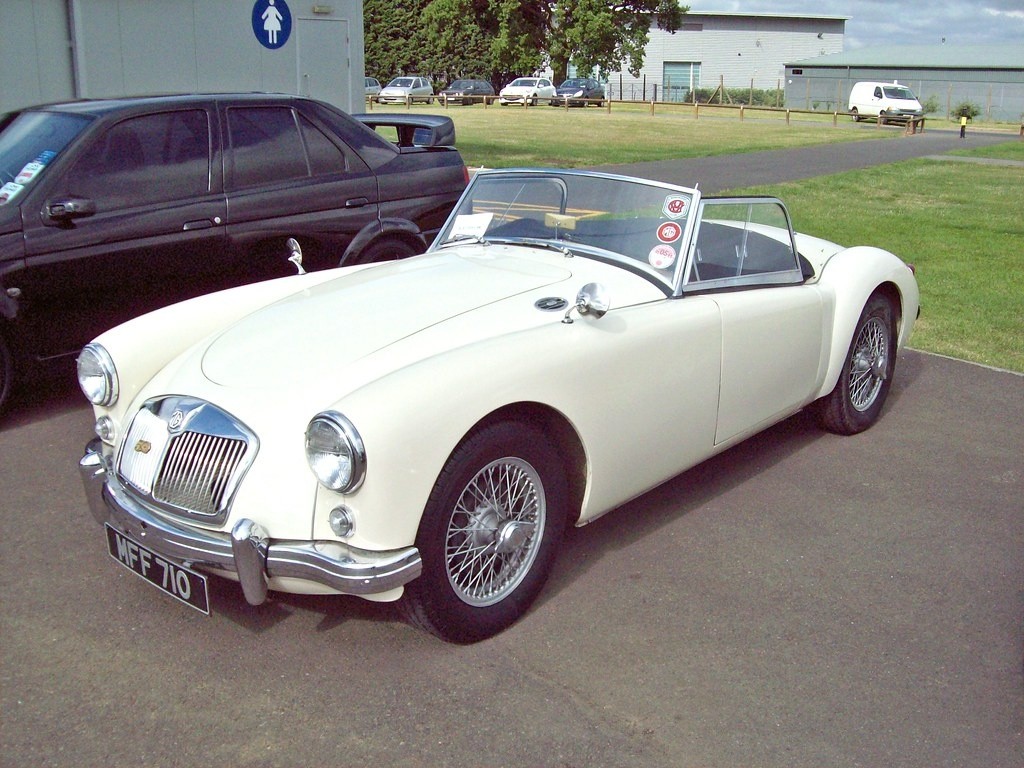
[365,77,382,103]
[77,169,920,644]
[551,78,606,107]
[1,90,473,417]
[437,80,495,106]
[378,77,434,105]
[499,78,557,106]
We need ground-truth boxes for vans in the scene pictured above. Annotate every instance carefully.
[848,82,923,126]
